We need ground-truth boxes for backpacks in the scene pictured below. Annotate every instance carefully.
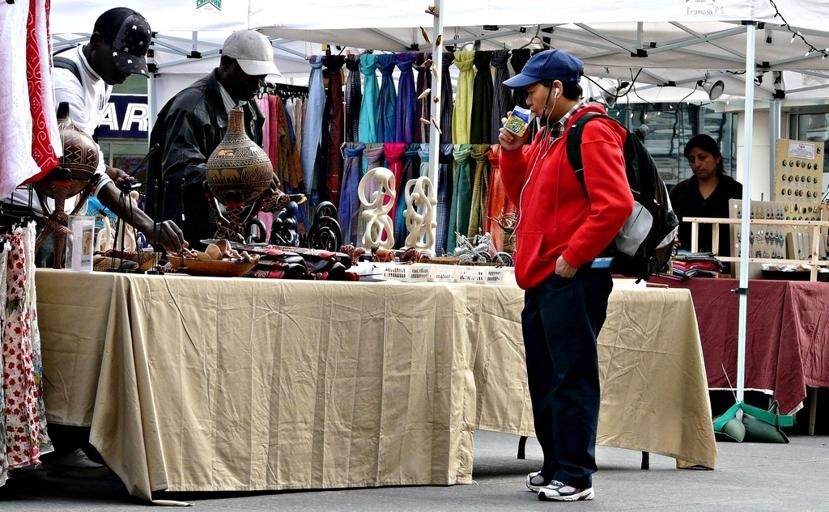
[566,112,679,284]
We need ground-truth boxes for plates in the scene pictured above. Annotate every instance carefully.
[165,253,261,276]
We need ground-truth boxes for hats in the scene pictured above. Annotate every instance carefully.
[94,8,150,78]
[502,49,584,89]
[221,30,282,77]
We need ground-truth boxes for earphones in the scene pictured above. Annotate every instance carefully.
[555,88,560,95]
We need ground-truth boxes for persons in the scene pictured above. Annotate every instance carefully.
[0,7,190,479]
[496,48,637,501]
[669,134,743,275]
[145,29,281,267]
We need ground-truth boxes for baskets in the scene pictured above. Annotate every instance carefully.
[356,262,517,285]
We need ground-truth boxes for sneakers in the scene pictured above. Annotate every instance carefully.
[526,471,544,493]
[538,480,595,502]
[60,448,104,469]
